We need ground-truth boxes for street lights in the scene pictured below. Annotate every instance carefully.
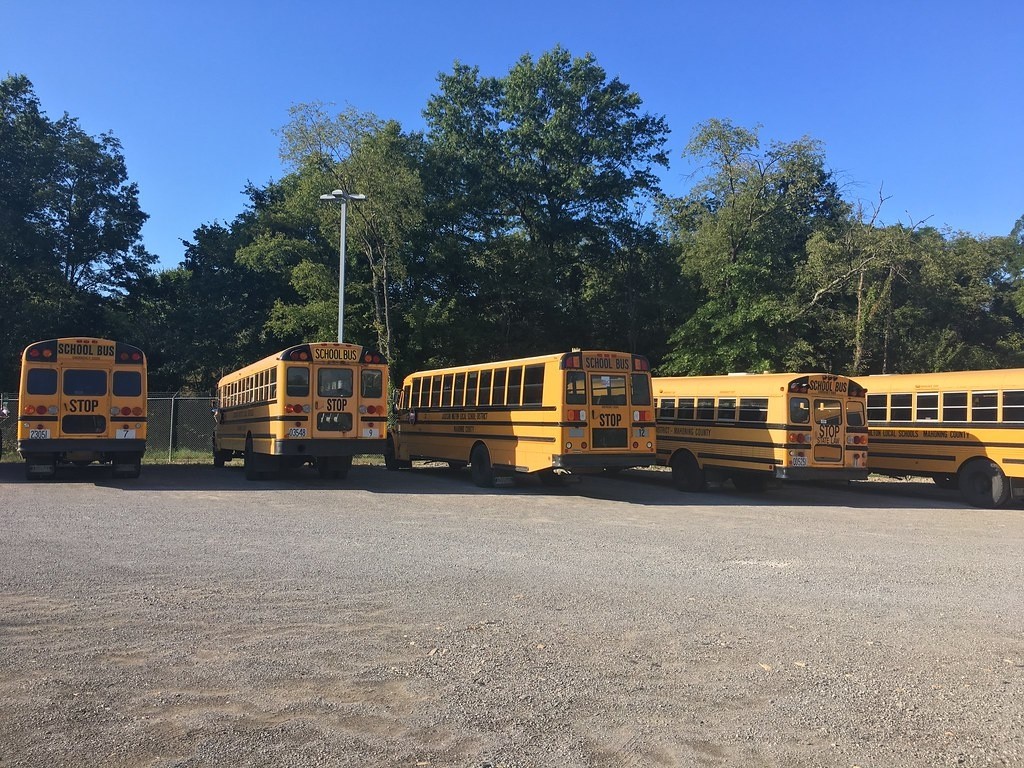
[318,186,368,344]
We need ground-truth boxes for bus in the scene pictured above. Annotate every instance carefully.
[834,368,1024,509]
[209,342,391,482]
[17,335,148,480]
[383,349,659,486]
[568,371,869,488]
